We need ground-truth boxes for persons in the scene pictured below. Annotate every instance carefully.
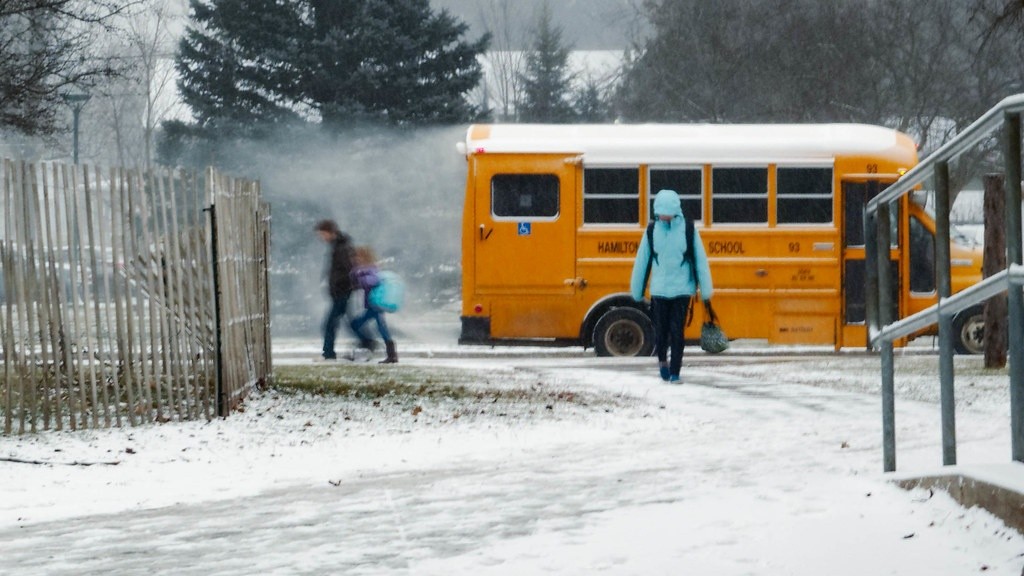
[314,218,398,364]
[631,190,712,384]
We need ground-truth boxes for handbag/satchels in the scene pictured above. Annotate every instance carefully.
[346,288,368,323]
[700,299,729,353]
[368,270,405,313]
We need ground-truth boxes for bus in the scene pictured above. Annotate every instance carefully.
[455,123,984,358]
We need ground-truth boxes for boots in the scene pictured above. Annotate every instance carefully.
[362,340,378,351]
[378,342,398,363]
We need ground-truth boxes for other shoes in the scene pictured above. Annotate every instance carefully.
[659,362,671,380]
[670,375,682,384]
[313,355,337,362]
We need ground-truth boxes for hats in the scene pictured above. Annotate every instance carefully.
[315,220,339,234]
[348,246,370,262]
